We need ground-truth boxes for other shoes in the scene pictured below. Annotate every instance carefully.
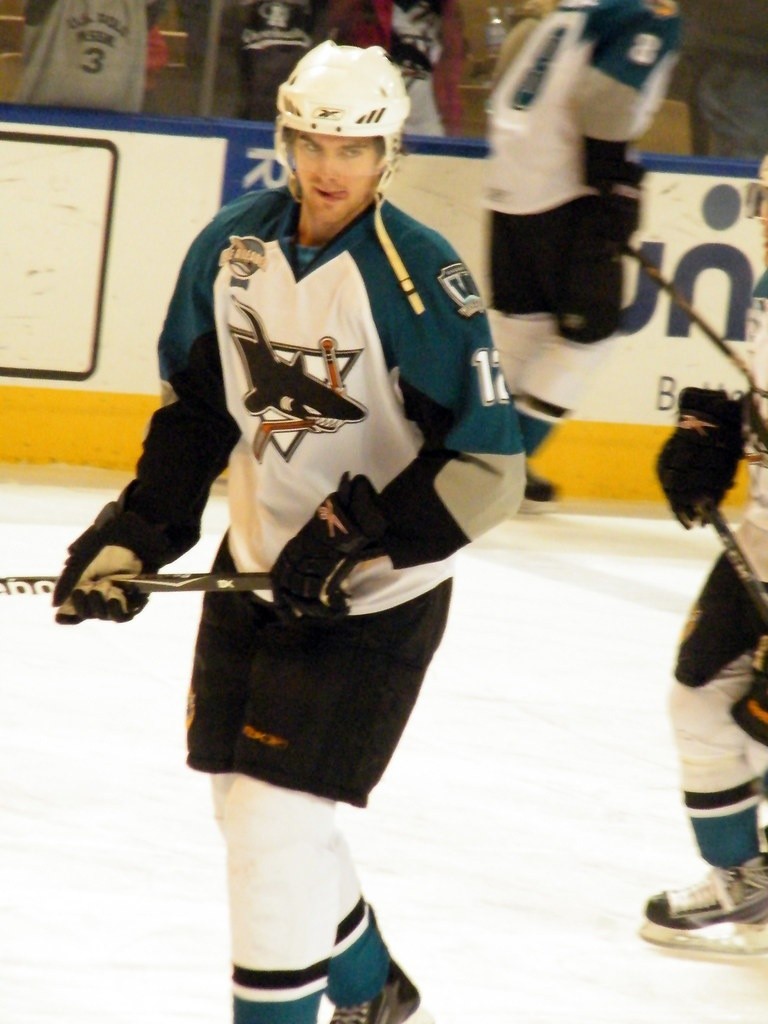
[516,462,556,515]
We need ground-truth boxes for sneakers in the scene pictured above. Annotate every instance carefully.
[636,852,768,955]
[329,955,434,1024]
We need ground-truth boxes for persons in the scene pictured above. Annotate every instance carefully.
[52,40,525,1024]
[644,153,768,930]
[9,0,467,138]
[488,0,686,501]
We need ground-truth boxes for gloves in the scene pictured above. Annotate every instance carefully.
[656,387,744,530]
[53,501,185,626]
[271,471,393,629]
[591,160,644,256]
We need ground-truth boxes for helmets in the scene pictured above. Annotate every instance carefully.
[742,154,768,221]
[275,40,410,180]
